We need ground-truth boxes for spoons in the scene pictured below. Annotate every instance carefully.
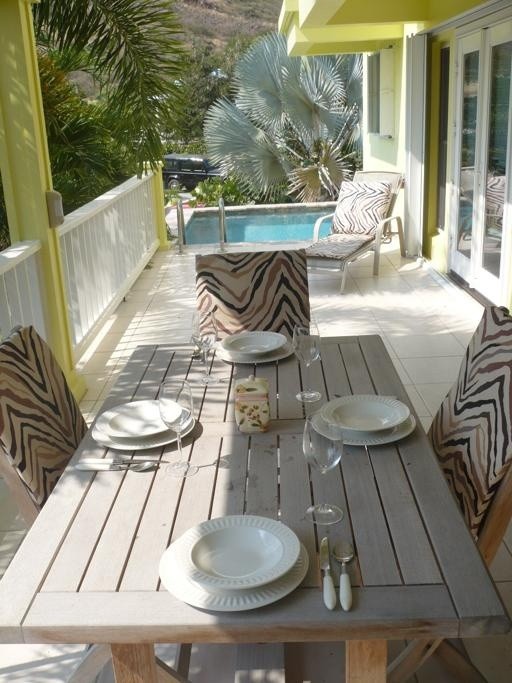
[333,535,357,613]
[71,459,154,473]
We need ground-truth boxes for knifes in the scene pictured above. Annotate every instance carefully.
[315,533,339,612]
[78,456,167,463]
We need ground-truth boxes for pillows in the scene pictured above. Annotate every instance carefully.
[331,177,391,234]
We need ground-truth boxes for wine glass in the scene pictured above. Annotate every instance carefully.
[191,310,218,385]
[291,323,325,402]
[156,377,198,477]
[301,412,345,525]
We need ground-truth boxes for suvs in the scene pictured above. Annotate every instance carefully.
[161,154,229,191]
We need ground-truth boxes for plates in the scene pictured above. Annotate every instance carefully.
[215,330,296,368]
[92,398,196,451]
[158,513,314,615]
[307,394,417,450]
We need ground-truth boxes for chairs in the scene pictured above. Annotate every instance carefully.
[1,320,91,508]
[193,247,312,342]
[426,301,512,572]
[304,170,408,295]
